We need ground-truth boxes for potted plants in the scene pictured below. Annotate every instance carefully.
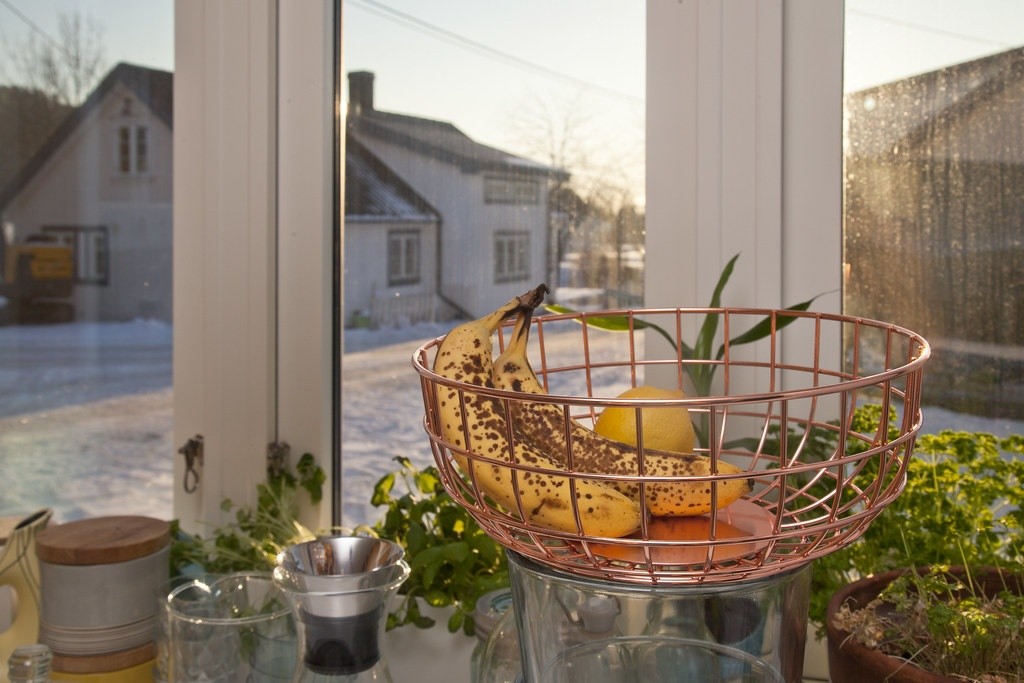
[169,451,509,683]
[762,402,1024,683]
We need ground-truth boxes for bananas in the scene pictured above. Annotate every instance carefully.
[431,282,754,540]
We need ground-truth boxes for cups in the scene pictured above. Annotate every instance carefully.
[151,569,307,683]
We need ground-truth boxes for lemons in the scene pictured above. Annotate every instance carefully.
[594,386,696,455]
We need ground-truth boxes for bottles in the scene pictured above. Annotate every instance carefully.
[5,644,54,683]
[270,559,412,683]
[501,551,809,683]
